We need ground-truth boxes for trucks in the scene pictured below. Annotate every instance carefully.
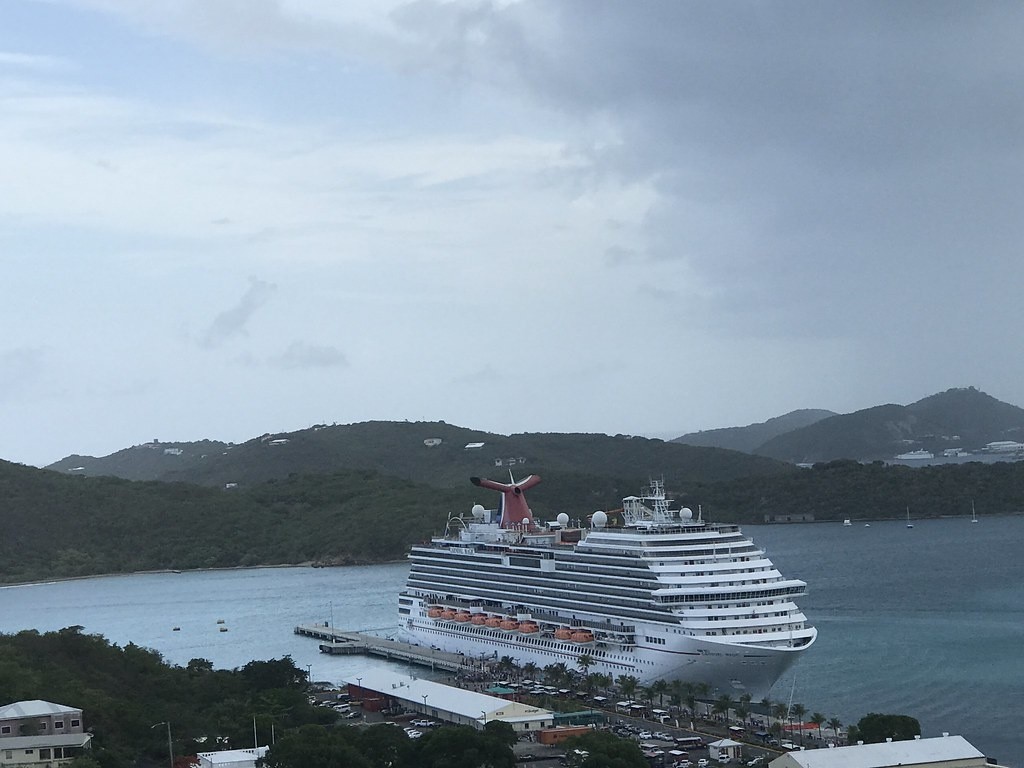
[641,744,663,758]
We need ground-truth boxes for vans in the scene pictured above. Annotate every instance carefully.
[674,736,706,750]
[333,704,350,713]
[338,694,350,701]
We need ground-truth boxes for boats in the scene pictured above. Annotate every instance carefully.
[865,524,870,527]
[842,519,852,527]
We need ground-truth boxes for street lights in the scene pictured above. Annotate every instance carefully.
[356,677,361,702]
[306,664,312,685]
[422,695,428,733]
[151,722,174,768]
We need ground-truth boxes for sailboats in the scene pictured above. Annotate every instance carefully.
[905,506,914,528]
[972,501,978,523]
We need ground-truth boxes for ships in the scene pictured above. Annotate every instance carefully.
[398,467,818,704]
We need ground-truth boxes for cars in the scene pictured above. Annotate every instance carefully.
[346,711,360,719]
[662,734,674,742]
[410,718,441,728]
[697,759,709,766]
[719,754,730,764]
[308,696,336,709]
[404,727,422,739]
[680,760,690,766]
[652,732,663,739]
[639,732,652,740]
